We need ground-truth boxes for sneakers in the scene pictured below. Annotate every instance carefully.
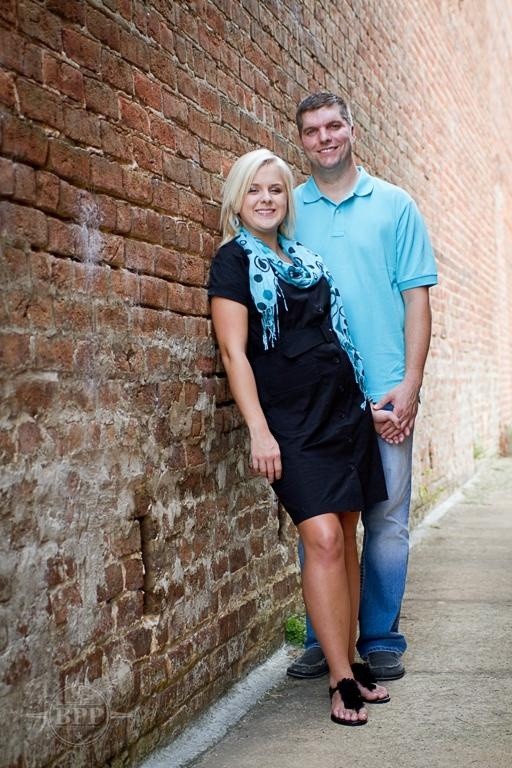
[286,647,329,678]
[366,652,404,681]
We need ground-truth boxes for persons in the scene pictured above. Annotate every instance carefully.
[278,92,440,683]
[207,148,410,727]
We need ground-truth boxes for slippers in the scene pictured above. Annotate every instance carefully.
[329,678,368,726]
[351,662,390,704]
